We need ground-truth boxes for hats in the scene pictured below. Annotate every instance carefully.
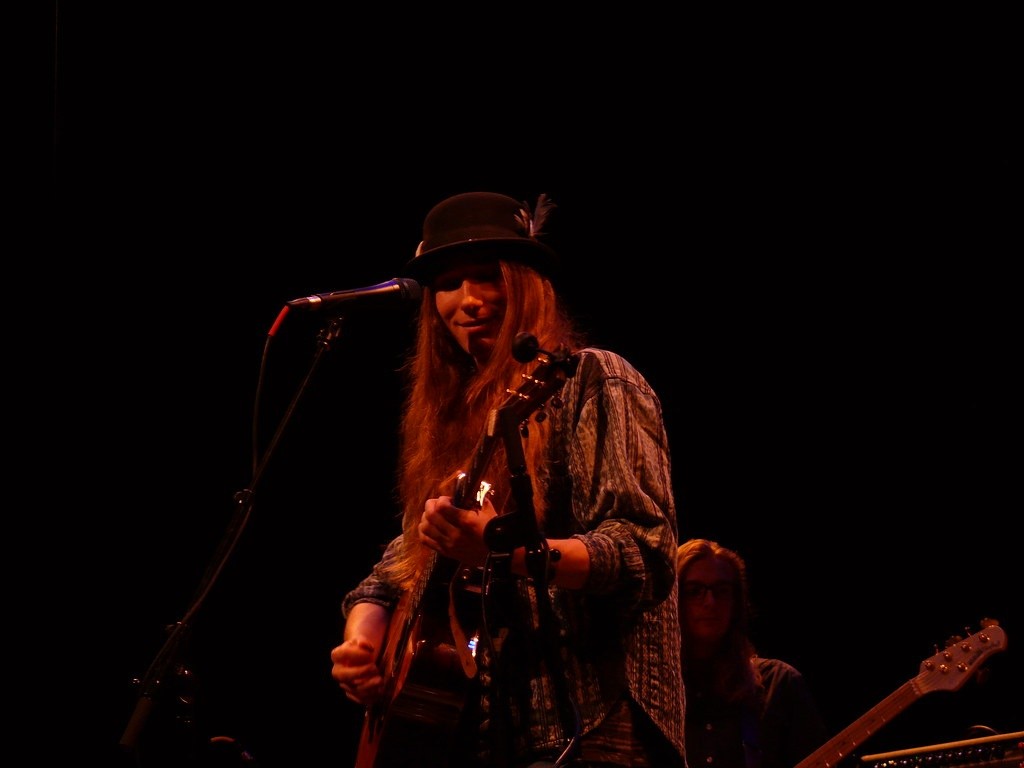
[406,194,545,264]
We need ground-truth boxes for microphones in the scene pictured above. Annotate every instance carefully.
[286,278,422,308]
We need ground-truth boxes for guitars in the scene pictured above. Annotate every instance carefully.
[352,332,582,767]
[789,614,1008,767]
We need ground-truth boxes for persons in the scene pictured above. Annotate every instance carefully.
[328,192,687,768]
[675,539,813,768]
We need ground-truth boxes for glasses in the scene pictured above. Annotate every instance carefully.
[678,578,739,603]
